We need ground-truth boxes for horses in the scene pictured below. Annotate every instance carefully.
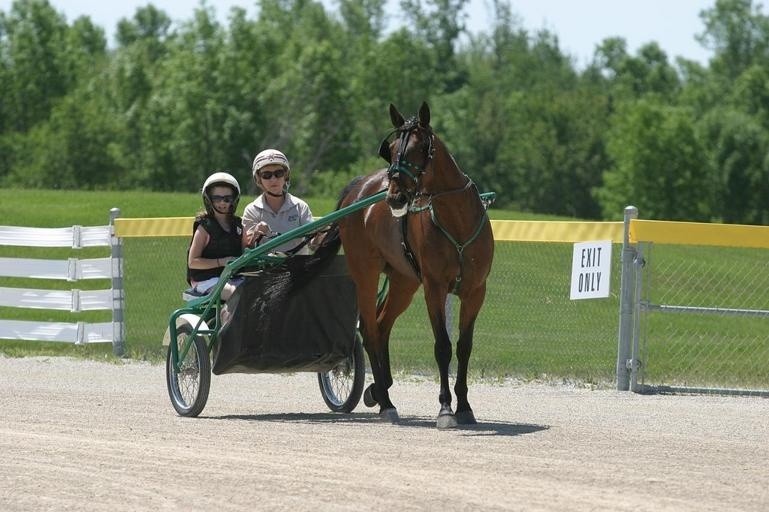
[253,101,496,430]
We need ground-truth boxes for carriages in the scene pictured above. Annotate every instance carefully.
[160,101,498,430]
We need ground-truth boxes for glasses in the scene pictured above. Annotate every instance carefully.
[261,169,285,179]
[211,194,234,203]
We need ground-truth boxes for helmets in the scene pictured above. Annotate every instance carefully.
[202,172,240,202]
[252,149,290,181]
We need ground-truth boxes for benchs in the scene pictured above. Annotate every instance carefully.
[181,288,226,309]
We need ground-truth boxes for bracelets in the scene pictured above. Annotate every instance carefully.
[217,258,221,267]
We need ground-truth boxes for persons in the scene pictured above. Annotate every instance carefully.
[241,148,331,256]
[186,171,248,302]
